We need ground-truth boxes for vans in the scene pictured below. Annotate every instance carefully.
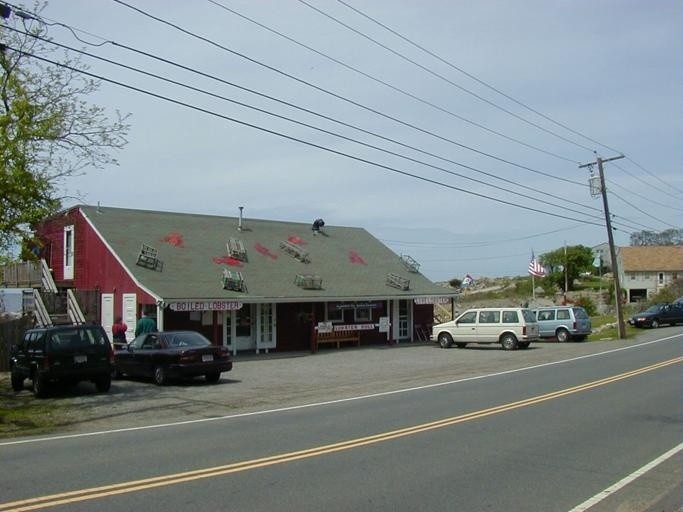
[529,305,591,344]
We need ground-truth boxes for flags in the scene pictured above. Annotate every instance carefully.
[527,258,545,279]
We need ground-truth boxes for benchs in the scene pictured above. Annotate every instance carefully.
[312,325,361,352]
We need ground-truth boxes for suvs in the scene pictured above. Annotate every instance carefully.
[429,306,540,350]
[10,320,117,398]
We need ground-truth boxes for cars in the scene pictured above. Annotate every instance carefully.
[113,328,233,386]
[627,296,682,329]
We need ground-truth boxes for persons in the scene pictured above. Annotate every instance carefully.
[136,309,156,337]
[112,317,127,343]
